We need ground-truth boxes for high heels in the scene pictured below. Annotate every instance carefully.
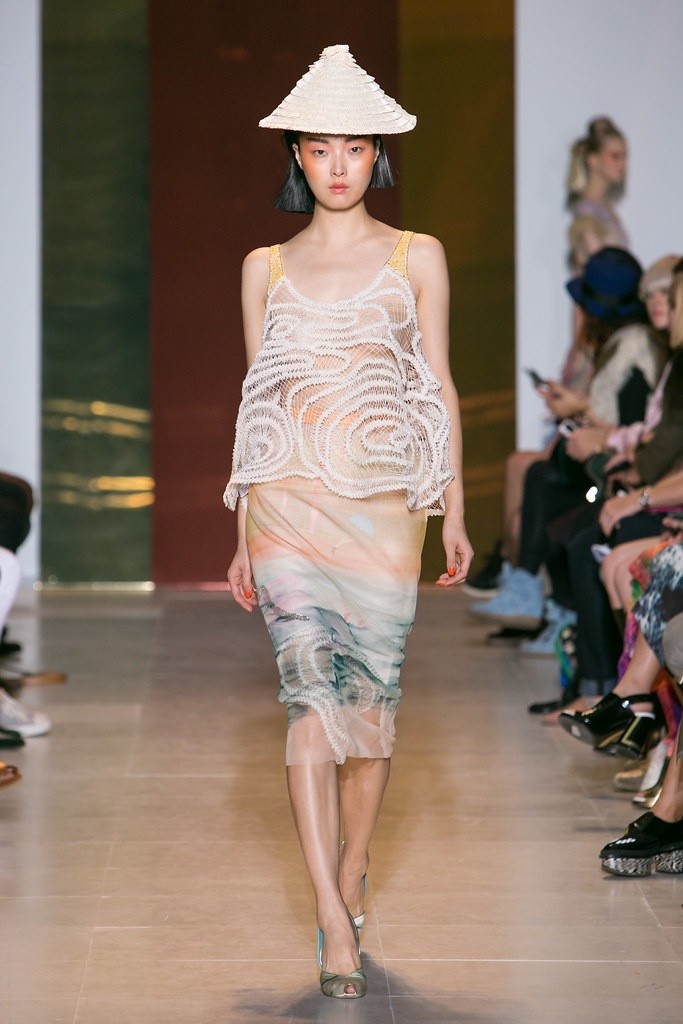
[317,916,367,999]
[598,812,683,876]
[530,672,674,809]
[339,841,370,929]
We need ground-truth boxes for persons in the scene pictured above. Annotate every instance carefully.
[0,472,68,786]
[464,114,682,880]
[223,42,475,999]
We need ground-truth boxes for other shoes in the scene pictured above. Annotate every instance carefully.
[0,639,66,787]
[461,553,578,655]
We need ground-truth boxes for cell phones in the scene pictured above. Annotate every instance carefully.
[560,418,576,437]
[527,369,551,393]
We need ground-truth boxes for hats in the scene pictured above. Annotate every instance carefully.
[256,45,417,135]
[566,249,649,321]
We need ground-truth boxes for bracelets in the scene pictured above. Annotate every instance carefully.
[639,488,651,508]
